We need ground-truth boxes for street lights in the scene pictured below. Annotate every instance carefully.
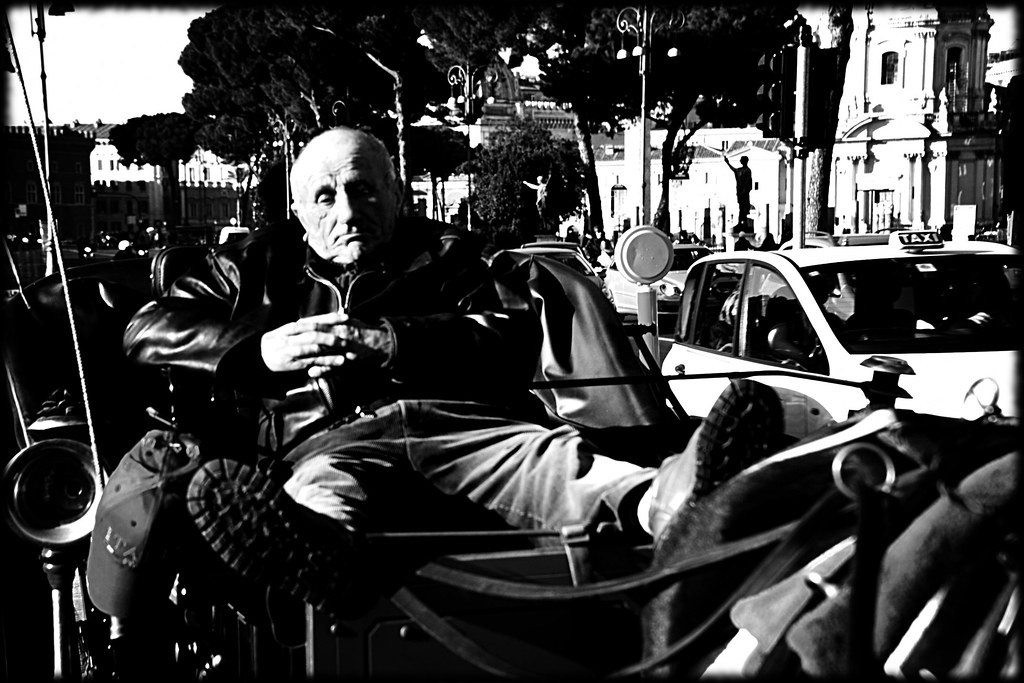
[448,60,502,230]
[614,0,689,224]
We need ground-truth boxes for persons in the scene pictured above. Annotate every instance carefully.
[522,174,550,233]
[566,221,778,268]
[121,126,788,637]
[724,155,752,219]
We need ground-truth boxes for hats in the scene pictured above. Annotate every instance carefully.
[86,430,201,618]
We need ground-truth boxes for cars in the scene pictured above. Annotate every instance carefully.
[658,229,1024,462]
[778,230,848,250]
[603,243,741,339]
[507,240,617,316]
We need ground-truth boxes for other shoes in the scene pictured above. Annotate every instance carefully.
[648,380,786,566]
[186,458,394,620]
